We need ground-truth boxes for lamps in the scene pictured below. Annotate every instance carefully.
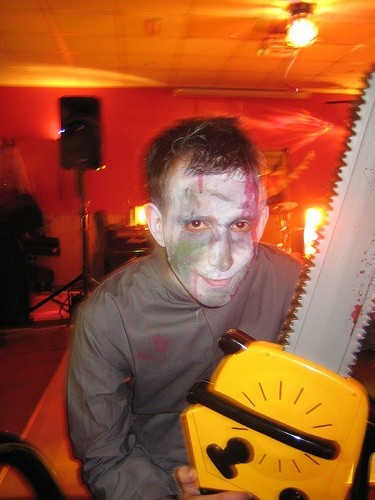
[285,2,318,48]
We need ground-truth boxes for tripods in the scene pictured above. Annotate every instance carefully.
[27,171,102,315]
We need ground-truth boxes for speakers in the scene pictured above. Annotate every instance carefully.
[58,95,101,170]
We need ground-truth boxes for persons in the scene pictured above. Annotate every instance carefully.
[64,113,313,500]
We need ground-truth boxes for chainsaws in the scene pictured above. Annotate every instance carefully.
[181,71,374,500]
